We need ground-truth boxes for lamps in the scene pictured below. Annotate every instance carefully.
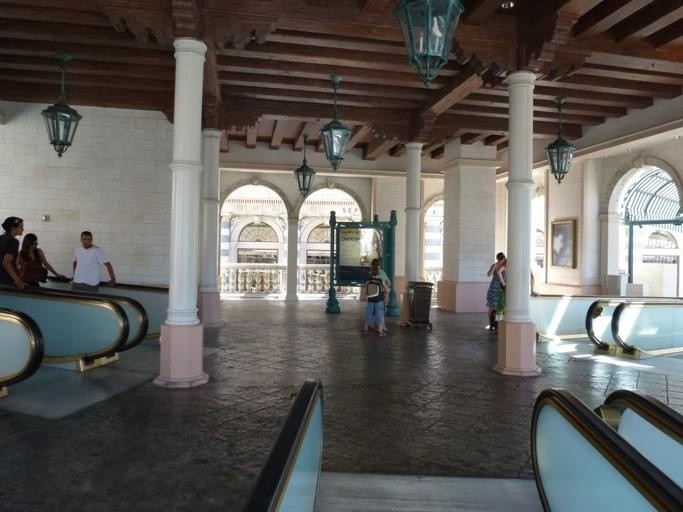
[394,0,466,92]
[294,73,350,198]
[544,97,576,185]
[39,51,83,158]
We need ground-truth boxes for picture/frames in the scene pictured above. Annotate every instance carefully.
[550,218,576,272]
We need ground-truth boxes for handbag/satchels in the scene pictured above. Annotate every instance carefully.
[37,268,48,283]
[490,310,497,326]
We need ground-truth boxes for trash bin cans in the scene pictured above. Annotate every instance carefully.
[405,281,434,330]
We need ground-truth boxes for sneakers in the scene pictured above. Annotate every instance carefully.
[362,328,387,336]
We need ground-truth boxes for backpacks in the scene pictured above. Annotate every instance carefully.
[365,278,384,302]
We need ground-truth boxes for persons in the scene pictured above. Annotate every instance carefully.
[362,265,392,336]
[69,231,116,292]
[369,258,391,330]
[485,252,540,329]
[16,233,66,287]
[0,216,30,289]
